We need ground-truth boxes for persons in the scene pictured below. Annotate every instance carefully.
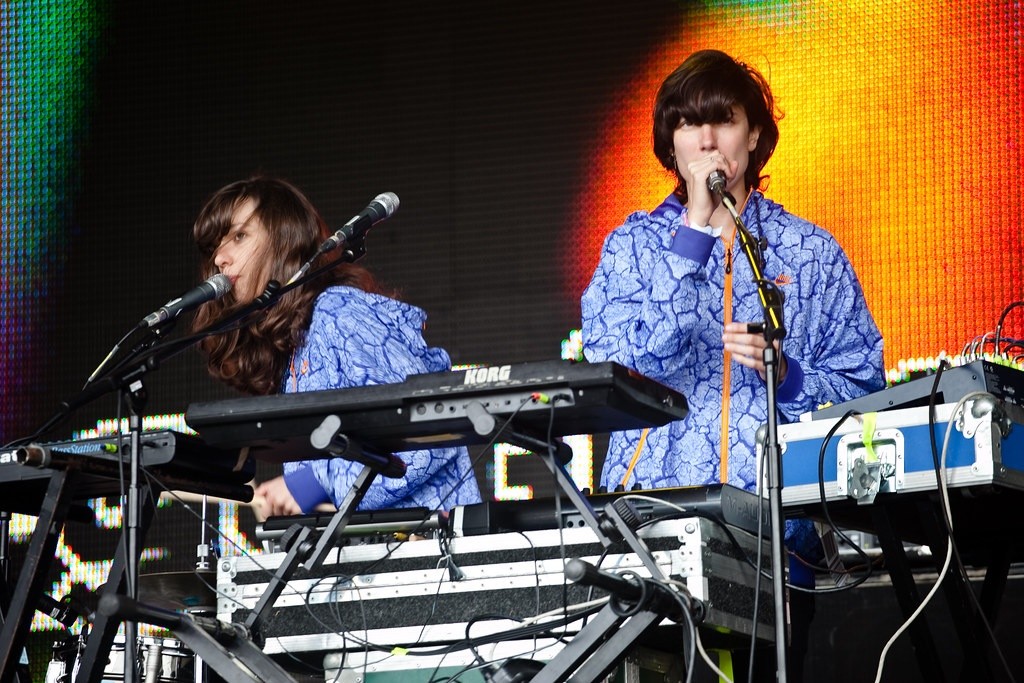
[580,49,888,683]
[194,174,483,521]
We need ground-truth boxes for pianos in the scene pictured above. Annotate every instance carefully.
[96,361,691,678]
[0,426,260,678]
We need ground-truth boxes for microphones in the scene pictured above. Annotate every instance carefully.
[140,273,233,327]
[706,170,727,192]
[320,192,400,252]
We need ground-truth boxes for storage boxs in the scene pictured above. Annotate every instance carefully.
[216,516,791,657]
[762,395,1024,566]
